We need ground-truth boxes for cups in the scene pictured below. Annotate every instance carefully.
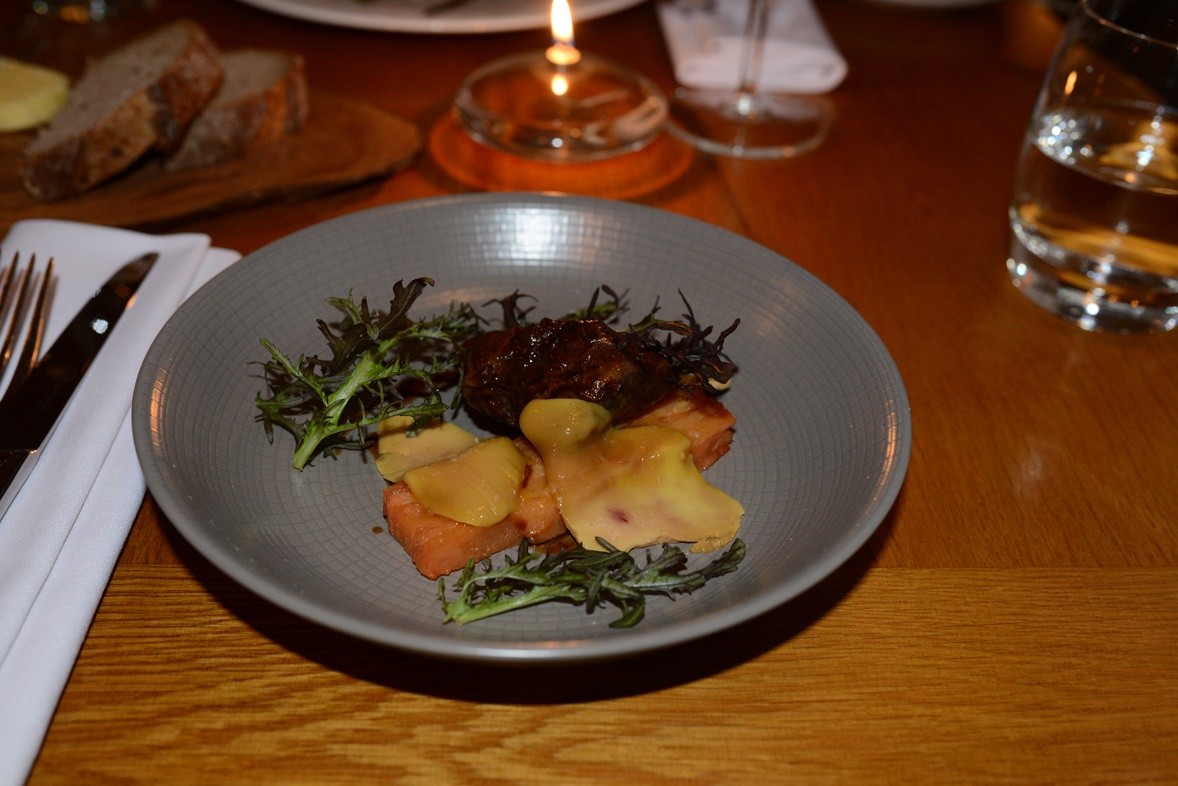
[1002,1,1177,339]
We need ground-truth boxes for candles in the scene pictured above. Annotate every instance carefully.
[542,1,583,75]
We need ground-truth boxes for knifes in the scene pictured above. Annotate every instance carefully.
[1,250,160,536]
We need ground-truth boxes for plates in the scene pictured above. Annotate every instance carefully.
[426,110,692,205]
[1,69,425,248]
[242,0,642,39]
[134,191,913,669]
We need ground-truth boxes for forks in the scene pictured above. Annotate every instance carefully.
[0,246,59,402]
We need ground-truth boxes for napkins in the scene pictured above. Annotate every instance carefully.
[0,218,240,786]
